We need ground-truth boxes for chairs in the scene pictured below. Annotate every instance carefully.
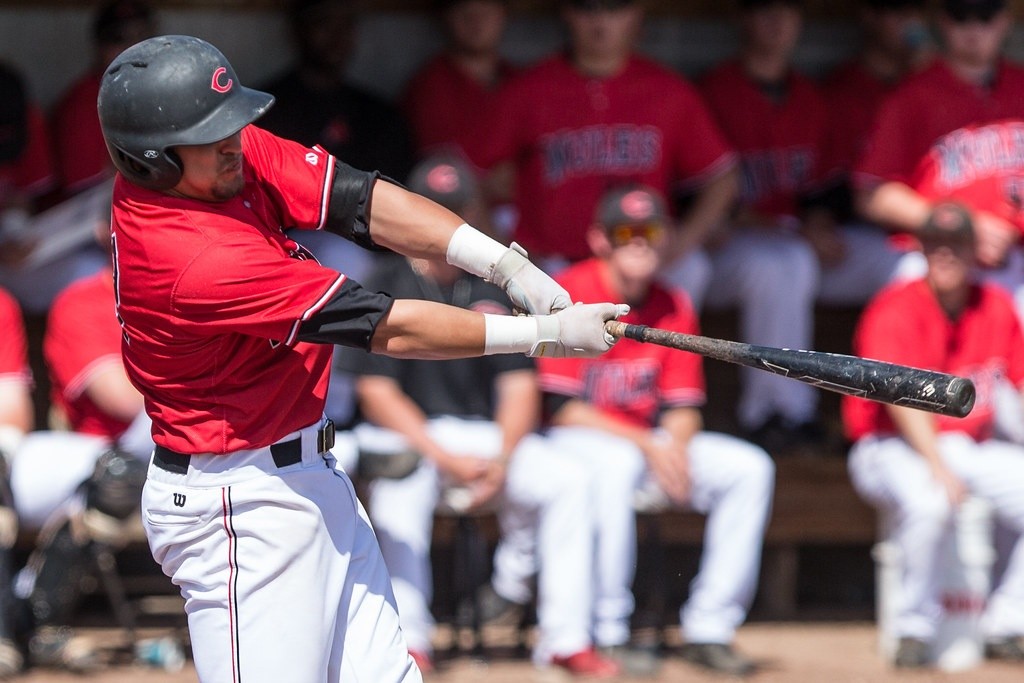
[91,504,670,661]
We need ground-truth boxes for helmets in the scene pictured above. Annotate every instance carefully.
[98,34,275,191]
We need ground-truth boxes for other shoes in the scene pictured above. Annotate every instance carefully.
[902,635,986,674]
[557,647,620,683]
[672,638,759,676]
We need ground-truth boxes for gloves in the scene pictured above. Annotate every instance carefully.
[486,238,573,314]
[524,301,630,361]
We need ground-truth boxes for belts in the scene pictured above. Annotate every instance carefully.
[149,418,337,470]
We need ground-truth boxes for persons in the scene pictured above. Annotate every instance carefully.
[0,0,1024,683]
[838,200,1024,670]
[532,183,778,677]
[96,33,631,683]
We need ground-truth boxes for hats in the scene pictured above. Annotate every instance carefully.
[409,157,491,231]
[600,184,666,229]
[917,207,973,243]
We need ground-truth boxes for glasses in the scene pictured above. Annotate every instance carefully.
[609,225,671,251]
[951,11,994,27]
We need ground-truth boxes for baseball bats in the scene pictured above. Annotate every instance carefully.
[508,306,977,418]
